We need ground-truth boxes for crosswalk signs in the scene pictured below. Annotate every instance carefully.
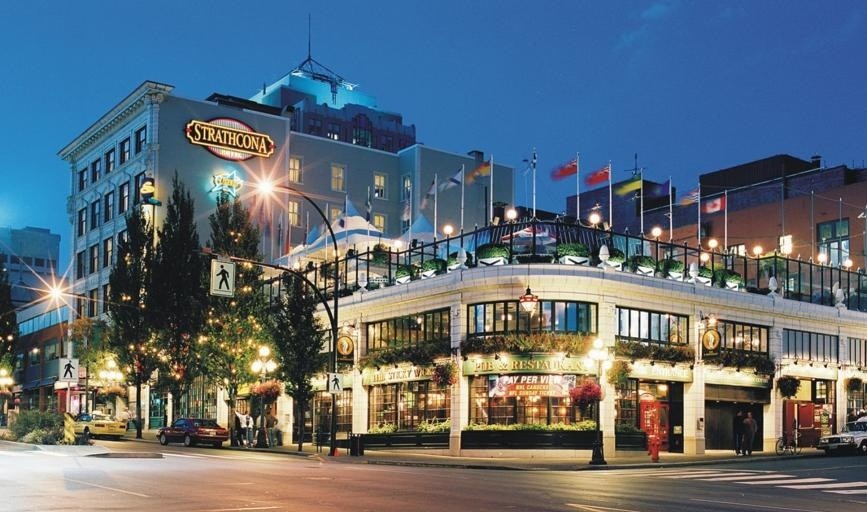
[328,372,343,394]
[58,358,79,382]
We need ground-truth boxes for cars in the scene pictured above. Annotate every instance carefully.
[817,416,867,455]
[156,418,229,448]
[74,411,126,440]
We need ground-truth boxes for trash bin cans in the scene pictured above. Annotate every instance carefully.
[349,433,364,455]
[7,409,16,428]
[695,437,704,454]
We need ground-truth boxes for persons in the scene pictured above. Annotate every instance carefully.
[733,410,758,457]
[234,410,279,449]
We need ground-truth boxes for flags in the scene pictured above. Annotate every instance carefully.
[613,175,641,196]
[420,158,491,210]
[550,159,577,181]
[584,166,609,187]
[700,196,725,215]
[679,188,699,206]
[646,179,670,200]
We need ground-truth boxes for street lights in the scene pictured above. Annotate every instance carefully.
[588,338,608,465]
[51,289,89,445]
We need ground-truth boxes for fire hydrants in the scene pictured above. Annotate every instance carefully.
[650,437,660,461]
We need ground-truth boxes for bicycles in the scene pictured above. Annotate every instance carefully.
[775,431,802,455]
